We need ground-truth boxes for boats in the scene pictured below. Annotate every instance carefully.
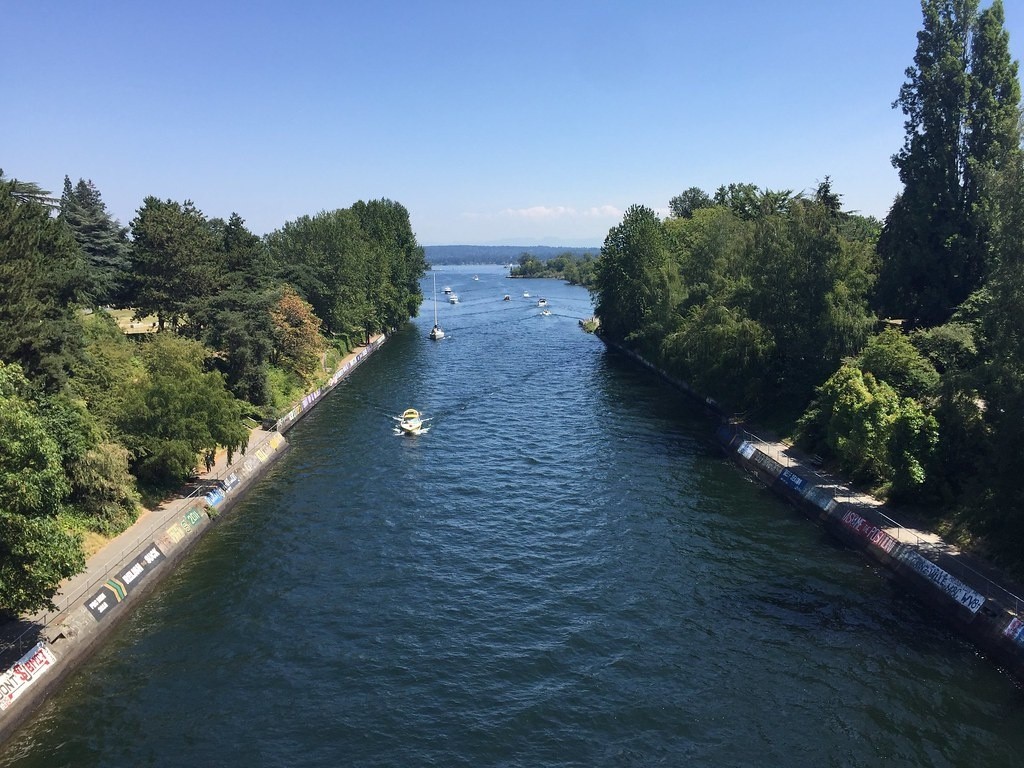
[523,290,530,298]
[449,294,458,304]
[503,294,511,301]
[542,308,551,316]
[444,285,451,295]
[399,408,421,436]
[537,298,547,306]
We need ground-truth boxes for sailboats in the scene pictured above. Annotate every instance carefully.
[428,271,445,340]
